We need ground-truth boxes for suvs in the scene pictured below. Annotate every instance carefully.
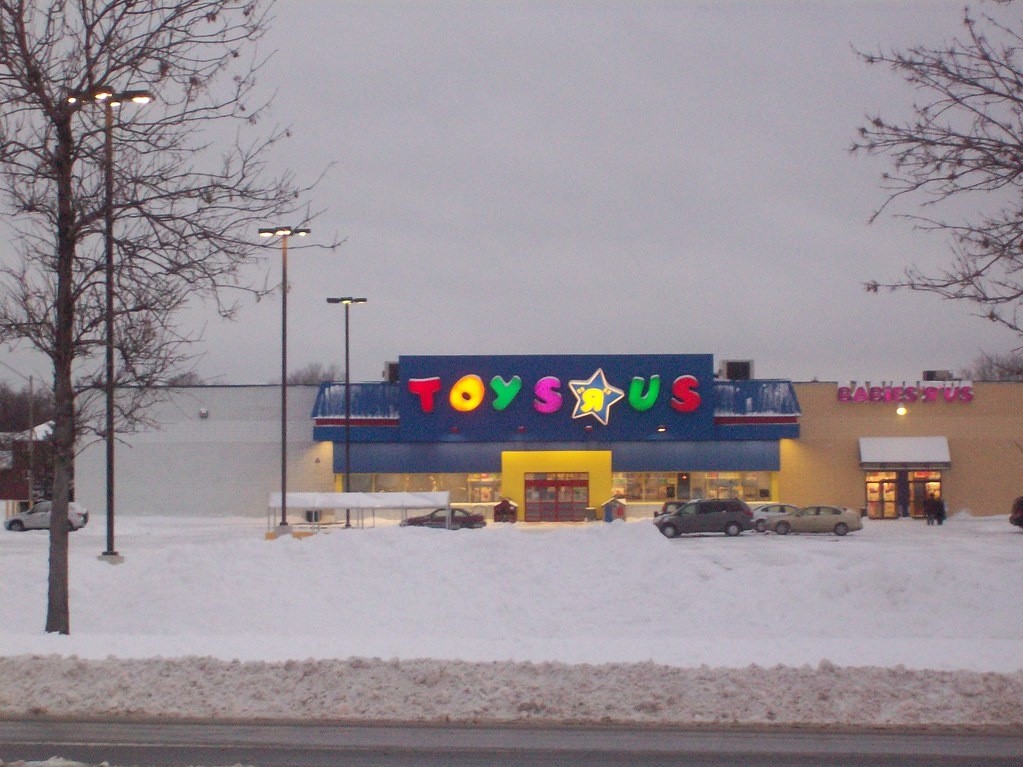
[653,498,754,538]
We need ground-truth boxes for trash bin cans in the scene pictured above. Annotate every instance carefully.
[585,507,597,521]
[306,511,318,522]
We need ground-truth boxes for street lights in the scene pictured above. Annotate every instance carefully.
[327,296,367,528]
[67,84,155,554]
[259,227,310,526]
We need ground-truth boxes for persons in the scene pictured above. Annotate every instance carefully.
[924,493,945,525]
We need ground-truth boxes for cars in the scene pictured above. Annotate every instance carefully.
[1009,496,1023,528]
[400,506,486,530]
[762,505,863,535]
[654,501,689,516]
[753,503,801,532]
[4,501,88,532]
